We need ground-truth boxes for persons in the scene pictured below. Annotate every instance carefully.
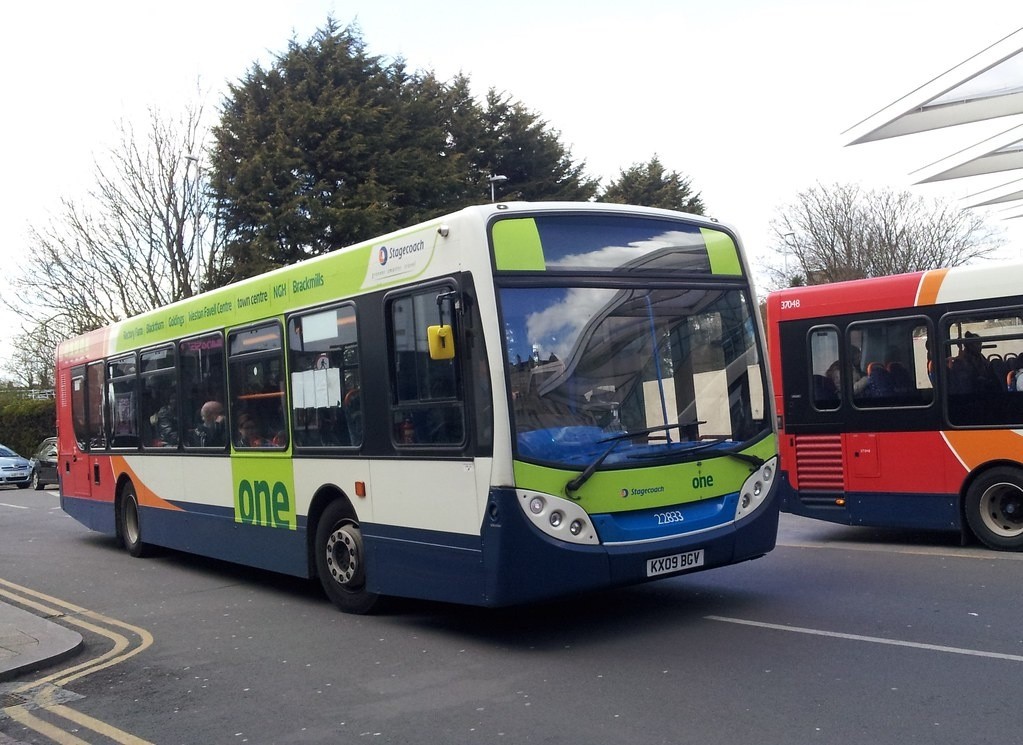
[926,331,1023,392]
[78,329,645,455]
[826,344,874,401]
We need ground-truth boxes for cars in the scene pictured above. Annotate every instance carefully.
[0,444,30,489]
[29,437,58,490]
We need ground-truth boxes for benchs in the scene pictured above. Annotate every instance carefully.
[814,372,867,404]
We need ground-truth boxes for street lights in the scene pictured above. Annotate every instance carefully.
[490,175,507,202]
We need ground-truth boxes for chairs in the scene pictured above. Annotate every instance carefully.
[345,389,363,447]
[868,351,1019,396]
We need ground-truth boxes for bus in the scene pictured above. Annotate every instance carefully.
[56,201,779,607]
[765,264,1023,552]
